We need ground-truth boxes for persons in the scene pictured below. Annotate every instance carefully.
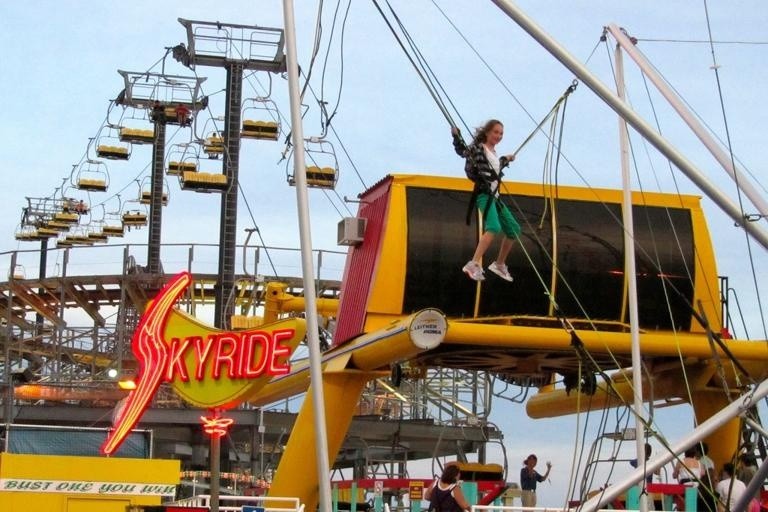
[208,132,218,159]
[672,441,761,512]
[520,454,553,512]
[452,119,522,283]
[425,464,473,512]
[630,443,661,483]
[151,100,168,126]
[174,103,192,128]
[219,132,224,154]
[63,198,89,216]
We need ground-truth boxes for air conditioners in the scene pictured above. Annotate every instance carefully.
[338,217,367,246]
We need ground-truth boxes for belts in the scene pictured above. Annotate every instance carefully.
[531,489,533,492]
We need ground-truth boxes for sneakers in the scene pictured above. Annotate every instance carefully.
[461,260,486,281]
[488,261,515,283]
